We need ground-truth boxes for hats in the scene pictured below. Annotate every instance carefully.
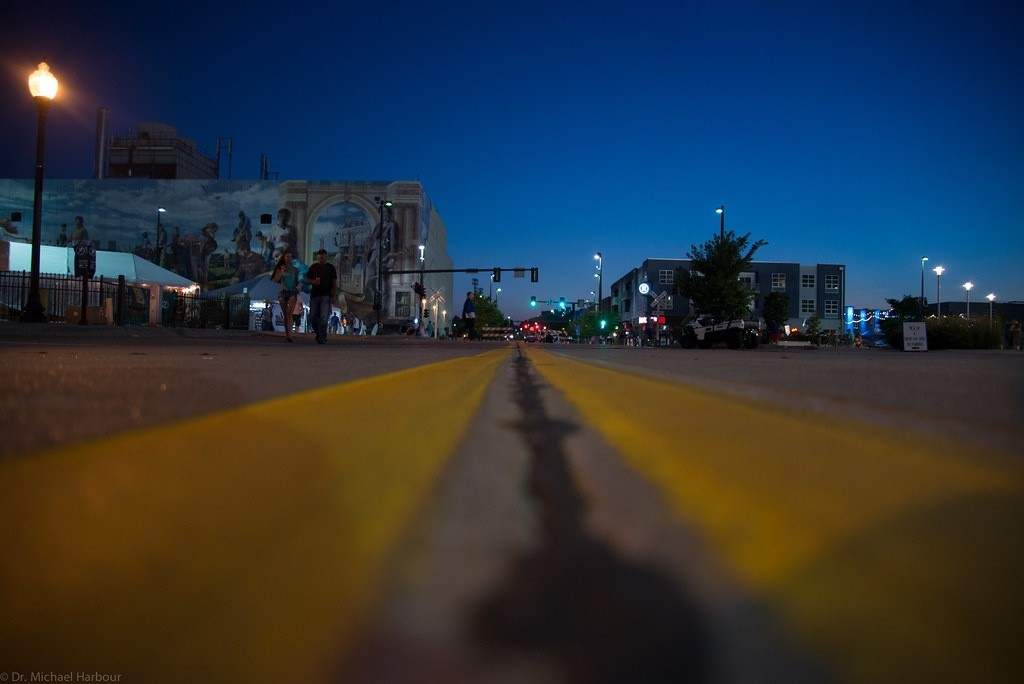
[316,249,327,255]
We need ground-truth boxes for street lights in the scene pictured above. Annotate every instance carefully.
[594,252,603,331]
[716,203,725,236]
[932,266,947,325]
[985,292,996,332]
[961,282,973,320]
[496,288,501,307]
[16,61,59,326]
[489,274,495,302]
[920,256,929,321]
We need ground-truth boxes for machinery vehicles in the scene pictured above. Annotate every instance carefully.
[678,313,762,350]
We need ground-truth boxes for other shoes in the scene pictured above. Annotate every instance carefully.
[315,335,325,344]
[286,337,292,343]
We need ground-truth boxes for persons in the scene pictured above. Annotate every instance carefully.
[271,251,298,342]
[307,249,338,343]
[293,283,303,337]
[327,311,362,337]
[462,292,476,340]
[260,301,273,330]
[426,321,435,337]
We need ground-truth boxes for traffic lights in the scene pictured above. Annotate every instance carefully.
[530,295,536,308]
[559,297,565,308]
[424,309,429,318]
